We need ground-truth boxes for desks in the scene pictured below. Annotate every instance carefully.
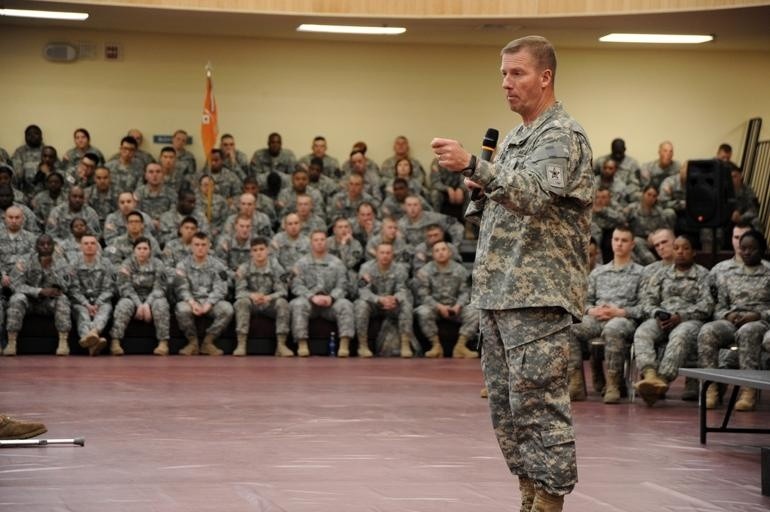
[678,369,770,500]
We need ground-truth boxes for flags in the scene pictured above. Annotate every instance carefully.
[200,78,219,157]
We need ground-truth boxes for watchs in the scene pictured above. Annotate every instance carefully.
[461,155,477,177]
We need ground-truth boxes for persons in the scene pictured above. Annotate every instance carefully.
[279,194,327,239]
[110,237,170,356]
[657,159,689,228]
[0,165,29,204]
[429,158,471,220]
[385,157,422,198]
[82,166,123,228]
[233,238,294,356]
[327,217,363,298]
[353,241,413,358]
[296,137,339,179]
[590,235,602,274]
[225,176,278,229]
[0,182,45,235]
[593,187,625,229]
[12,124,60,187]
[710,223,770,302]
[172,130,197,181]
[29,173,67,220]
[277,170,327,220]
[268,213,311,271]
[214,213,259,273]
[3,235,71,355]
[220,193,275,237]
[431,36,596,512]
[45,187,102,241]
[261,172,282,197]
[365,218,411,265]
[641,142,681,191]
[384,178,434,221]
[594,138,639,185]
[105,135,145,193]
[289,229,355,357]
[569,226,645,403]
[103,192,159,246]
[250,133,295,188]
[61,129,106,173]
[199,149,244,202]
[65,152,99,189]
[342,141,381,175]
[338,150,381,201]
[68,235,117,356]
[106,129,155,164]
[634,233,714,407]
[398,196,464,247]
[161,216,198,268]
[220,134,248,181]
[158,188,212,244]
[412,223,463,272]
[414,241,479,358]
[697,230,770,410]
[711,144,740,174]
[731,169,760,223]
[595,158,626,204]
[382,137,426,185]
[620,184,669,261]
[160,147,192,192]
[330,174,381,226]
[175,232,233,356]
[132,162,178,220]
[347,203,382,244]
[196,174,228,237]
[295,157,336,200]
[25,147,69,198]
[645,228,676,274]
[0,207,37,353]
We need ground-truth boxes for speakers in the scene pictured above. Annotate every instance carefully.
[683,158,734,227]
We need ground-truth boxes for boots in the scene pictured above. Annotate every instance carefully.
[297,339,310,357]
[153,340,169,356]
[337,336,350,357]
[631,375,669,407]
[3,331,17,355]
[110,338,124,356]
[400,334,413,358]
[681,377,699,400]
[200,333,224,356]
[601,376,628,397]
[425,335,444,358]
[232,334,247,356]
[636,367,669,394]
[56,331,70,356]
[78,328,99,348]
[452,335,479,358]
[530,489,564,512]
[734,386,756,411]
[705,383,722,408]
[357,336,373,357]
[519,477,535,512]
[568,369,585,400]
[0,414,48,441]
[481,387,488,398]
[179,335,200,355]
[80,333,107,356]
[275,334,294,357]
[603,369,621,403]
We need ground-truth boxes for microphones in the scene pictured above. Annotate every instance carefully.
[470,126,499,202]
